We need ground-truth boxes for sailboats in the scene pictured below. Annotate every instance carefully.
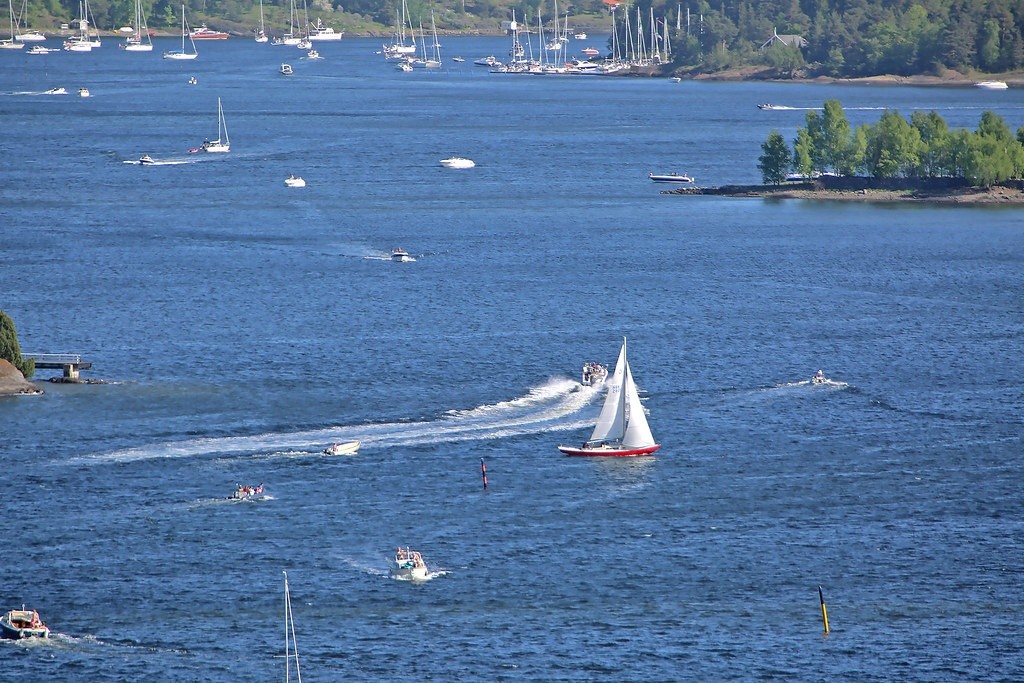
[1,0,676,100]
[557,336,662,458]
[202,96,231,154]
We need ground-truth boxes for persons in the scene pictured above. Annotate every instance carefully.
[333,443,336,451]
[583,441,589,448]
[397,547,421,568]
[817,369,822,375]
[244,483,263,492]
[39,622,46,629]
[588,361,602,375]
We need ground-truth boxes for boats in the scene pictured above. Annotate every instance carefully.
[809,369,832,386]
[784,168,845,182]
[224,482,268,501]
[387,545,428,580]
[322,440,362,457]
[649,170,693,184]
[581,361,609,387]
[284,174,306,188]
[0,601,50,642]
[391,246,409,262]
[972,80,1011,93]
[138,154,155,164]
[757,102,774,111]
[439,156,475,169]
